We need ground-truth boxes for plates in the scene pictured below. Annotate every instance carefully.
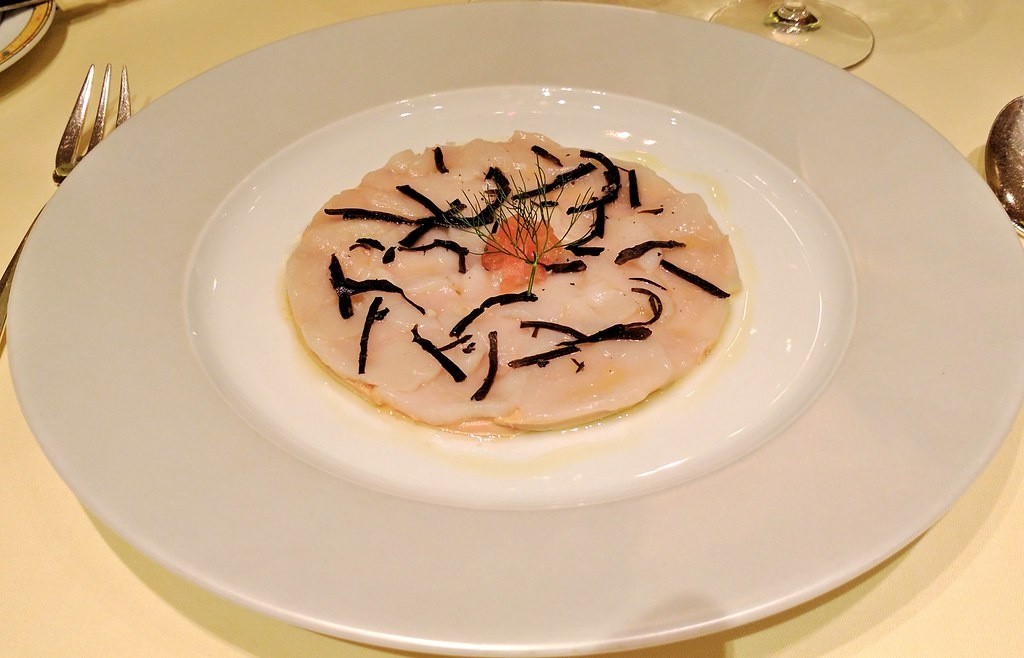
[0,1,56,73]
[7,2,1024,657]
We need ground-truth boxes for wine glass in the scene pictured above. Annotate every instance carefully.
[711,0,875,69]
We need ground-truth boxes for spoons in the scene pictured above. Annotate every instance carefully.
[986,95,1024,234]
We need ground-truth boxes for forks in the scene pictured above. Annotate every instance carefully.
[0,63,130,336]
